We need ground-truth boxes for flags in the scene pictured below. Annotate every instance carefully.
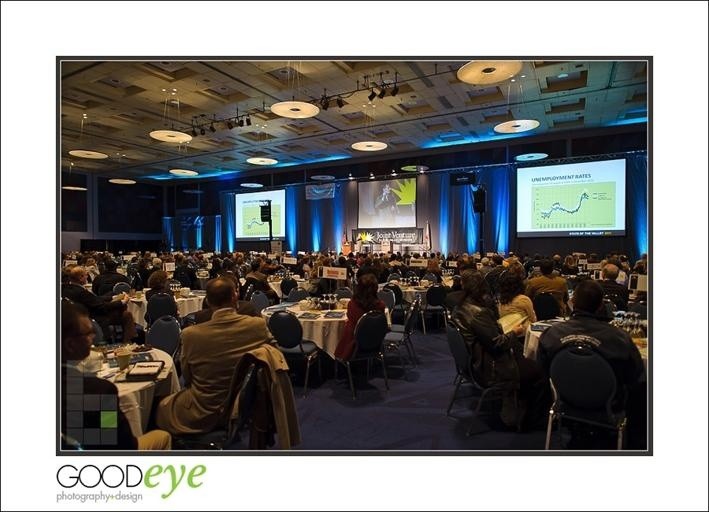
[425,223,431,251]
[341,225,347,250]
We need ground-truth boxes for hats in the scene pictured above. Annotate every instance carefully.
[461,269,483,292]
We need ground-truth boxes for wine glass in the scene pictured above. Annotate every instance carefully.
[275,272,294,279]
[169,284,182,291]
[442,269,454,276]
[352,268,358,279]
[322,293,338,305]
[612,310,646,348]
[306,296,322,311]
[399,276,419,285]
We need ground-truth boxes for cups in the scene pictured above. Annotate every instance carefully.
[117,351,130,370]
[136,291,142,299]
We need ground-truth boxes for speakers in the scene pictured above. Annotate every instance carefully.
[450,172,476,185]
[261,206,270,222]
[474,190,487,212]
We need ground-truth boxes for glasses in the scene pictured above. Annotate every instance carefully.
[74,326,97,338]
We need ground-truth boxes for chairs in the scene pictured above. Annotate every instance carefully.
[58,246,651,455]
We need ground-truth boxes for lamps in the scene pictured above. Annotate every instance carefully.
[62,60,550,192]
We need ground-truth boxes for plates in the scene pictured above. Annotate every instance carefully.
[131,346,156,355]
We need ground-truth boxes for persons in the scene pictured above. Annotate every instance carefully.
[372,183,399,216]
[355,232,367,253]
